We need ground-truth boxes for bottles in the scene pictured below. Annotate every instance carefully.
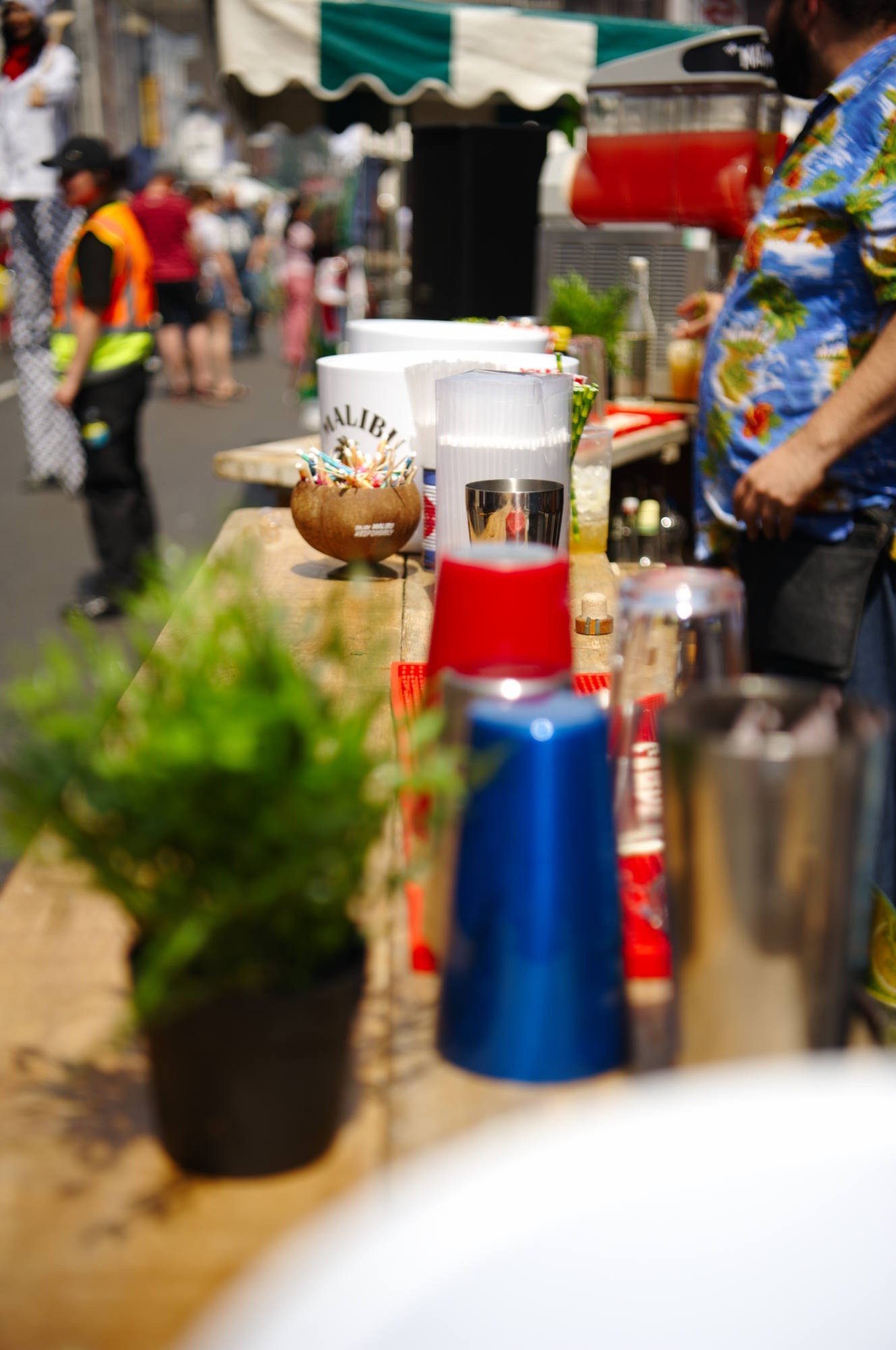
[612,256,655,400]
[420,537,637,1081]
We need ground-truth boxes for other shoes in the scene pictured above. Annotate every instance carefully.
[70,577,127,619]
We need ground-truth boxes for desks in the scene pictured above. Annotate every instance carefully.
[0,508,682,1349]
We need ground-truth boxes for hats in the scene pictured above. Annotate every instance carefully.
[17,0,48,17]
[43,137,116,174]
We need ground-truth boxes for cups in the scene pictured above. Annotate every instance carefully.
[655,673,869,1067]
[316,315,603,593]
[667,339,703,400]
[607,561,743,862]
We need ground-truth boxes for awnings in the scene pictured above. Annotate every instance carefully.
[217,2,775,126]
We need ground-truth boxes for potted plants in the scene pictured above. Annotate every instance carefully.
[2,532,401,1178]
[545,269,636,401]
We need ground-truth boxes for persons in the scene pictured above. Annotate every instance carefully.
[0,1,77,203]
[135,100,316,400]
[39,134,160,613]
[679,0,896,911]
